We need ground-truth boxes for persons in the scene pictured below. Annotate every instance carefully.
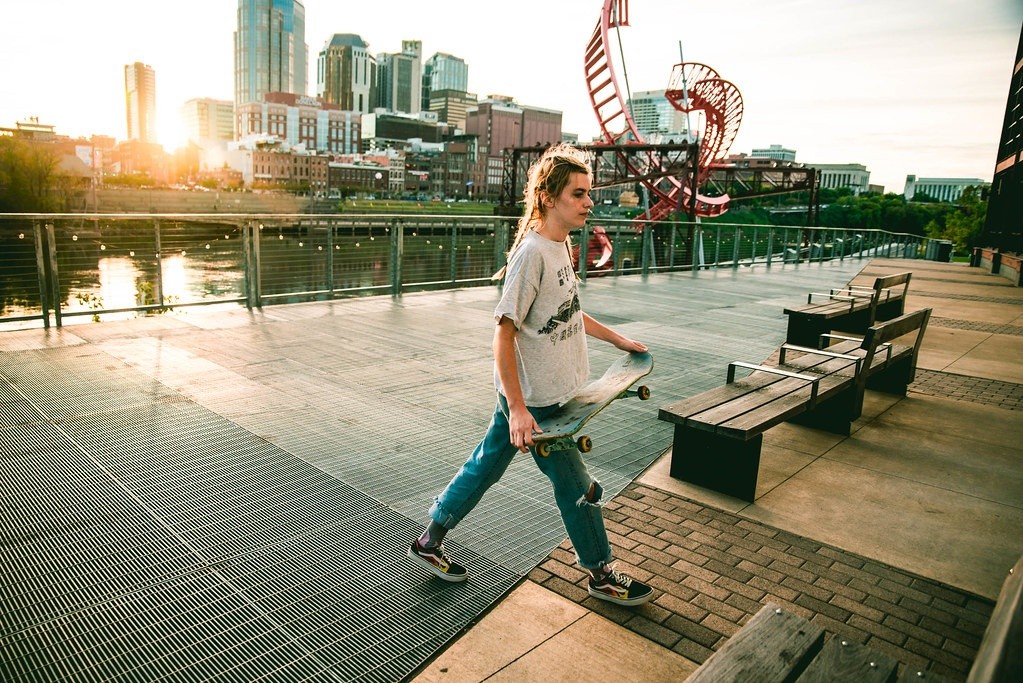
[406,154,654,607]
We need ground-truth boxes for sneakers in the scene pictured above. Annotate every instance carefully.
[588,563,653,606]
[406,537,467,582]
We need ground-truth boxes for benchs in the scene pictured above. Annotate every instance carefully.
[683,559,1023,683]
[781,271,914,352]
[657,305,933,506]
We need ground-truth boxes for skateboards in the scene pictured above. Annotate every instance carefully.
[522,348,654,458]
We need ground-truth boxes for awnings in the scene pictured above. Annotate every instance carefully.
[465,182,474,186]
